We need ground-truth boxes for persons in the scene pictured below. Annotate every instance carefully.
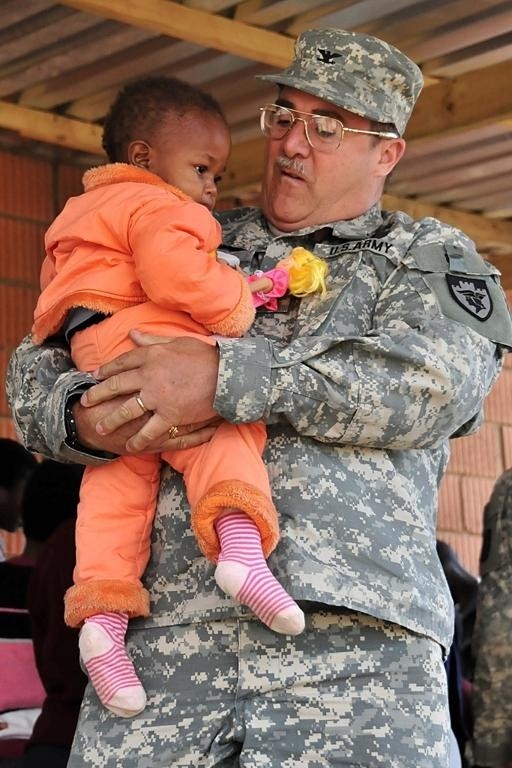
[233,245,331,309]
[31,66,309,721]
[4,24,511,767]
[468,467,512,768]
[0,437,89,768]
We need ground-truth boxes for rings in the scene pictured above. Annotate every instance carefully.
[133,393,149,412]
[167,425,181,441]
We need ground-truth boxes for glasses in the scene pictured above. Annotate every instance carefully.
[258,100,400,158]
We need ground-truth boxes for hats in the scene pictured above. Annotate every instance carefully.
[252,27,426,139]
